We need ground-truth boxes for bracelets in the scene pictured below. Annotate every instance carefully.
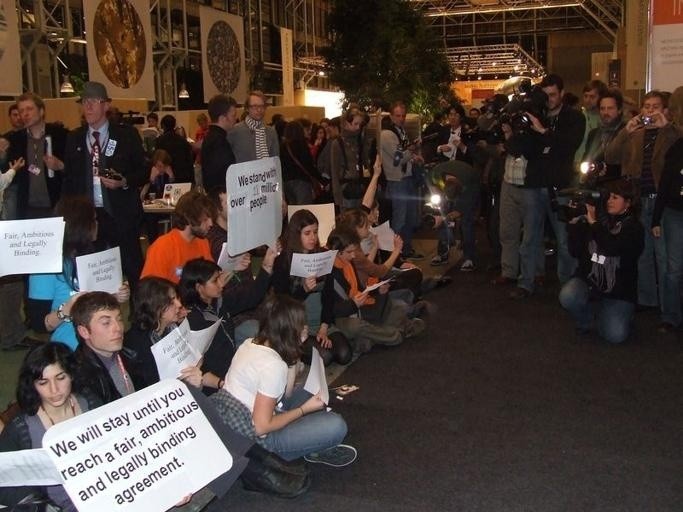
[296,405,305,417]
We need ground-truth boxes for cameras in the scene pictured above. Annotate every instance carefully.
[641,114,652,125]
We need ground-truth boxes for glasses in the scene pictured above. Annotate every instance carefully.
[645,105,660,111]
[251,105,266,110]
[448,114,459,118]
[81,98,109,105]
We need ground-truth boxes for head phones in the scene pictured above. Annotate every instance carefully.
[346,107,372,125]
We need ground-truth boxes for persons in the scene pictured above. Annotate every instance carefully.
[215,185,284,308]
[272,209,352,368]
[358,153,454,294]
[329,105,374,204]
[59,290,311,497]
[427,72,683,344]
[0,341,103,512]
[1,104,24,141]
[337,205,427,344]
[119,275,199,394]
[138,190,215,285]
[318,225,406,347]
[63,81,145,285]
[175,253,237,388]
[200,93,238,201]
[272,111,340,204]
[140,110,206,206]
[4,90,75,326]
[227,89,280,164]
[0,155,25,189]
[379,101,426,262]
[219,294,358,467]
[26,193,131,350]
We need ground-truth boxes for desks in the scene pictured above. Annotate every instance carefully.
[142,198,176,235]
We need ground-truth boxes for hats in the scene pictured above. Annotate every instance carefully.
[76,82,112,105]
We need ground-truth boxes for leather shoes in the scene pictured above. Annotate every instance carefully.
[243,464,311,498]
[266,453,313,479]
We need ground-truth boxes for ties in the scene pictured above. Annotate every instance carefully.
[92,131,101,152]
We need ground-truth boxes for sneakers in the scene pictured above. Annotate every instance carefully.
[490,276,510,286]
[460,259,479,272]
[404,255,424,261]
[508,287,527,299]
[410,300,431,315]
[432,256,449,266]
[404,318,425,339]
[304,444,357,467]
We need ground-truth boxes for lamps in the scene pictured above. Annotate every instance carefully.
[177,82,189,100]
[58,71,74,93]
